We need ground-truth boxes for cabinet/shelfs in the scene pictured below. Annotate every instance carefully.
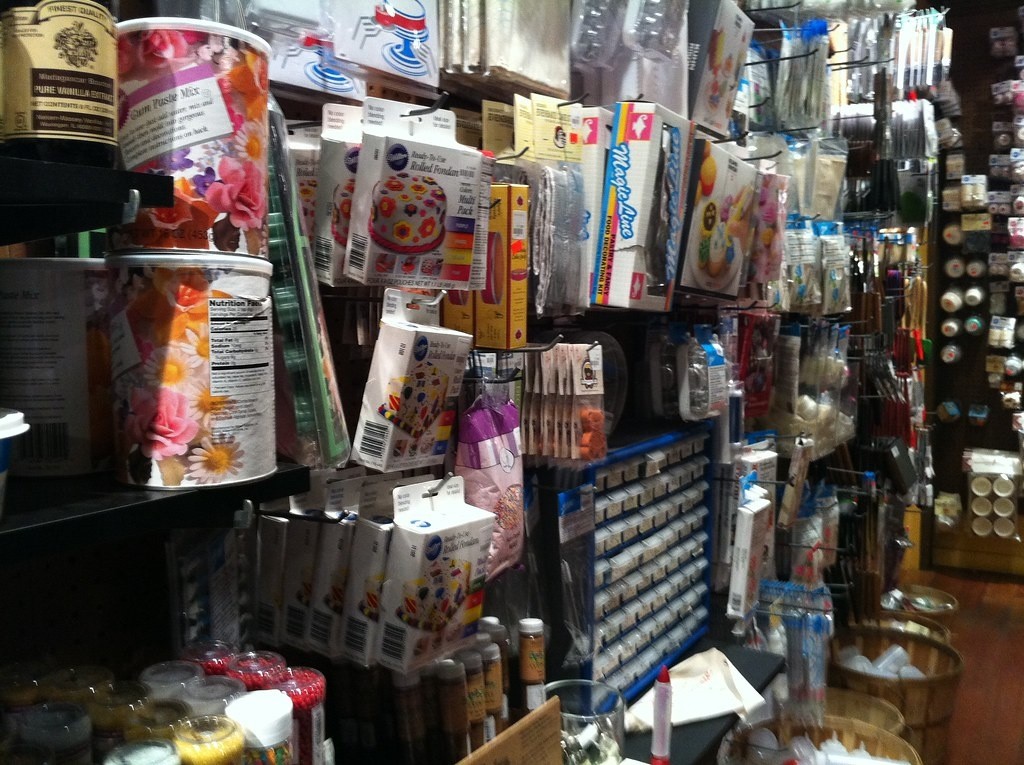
[0,0,313,560]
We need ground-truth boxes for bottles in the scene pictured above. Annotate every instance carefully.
[0,641,325,765]
[394,615,544,765]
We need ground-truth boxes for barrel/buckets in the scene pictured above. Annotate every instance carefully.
[828,629,964,765]
[772,689,907,742]
[717,716,922,765]
[850,611,951,649]
[879,584,960,629]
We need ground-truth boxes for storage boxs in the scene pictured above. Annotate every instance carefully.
[580,0,790,318]
[238,101,564,678]
[712,433,778,620]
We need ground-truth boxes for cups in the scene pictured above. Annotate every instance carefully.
[544,679,624,765]
